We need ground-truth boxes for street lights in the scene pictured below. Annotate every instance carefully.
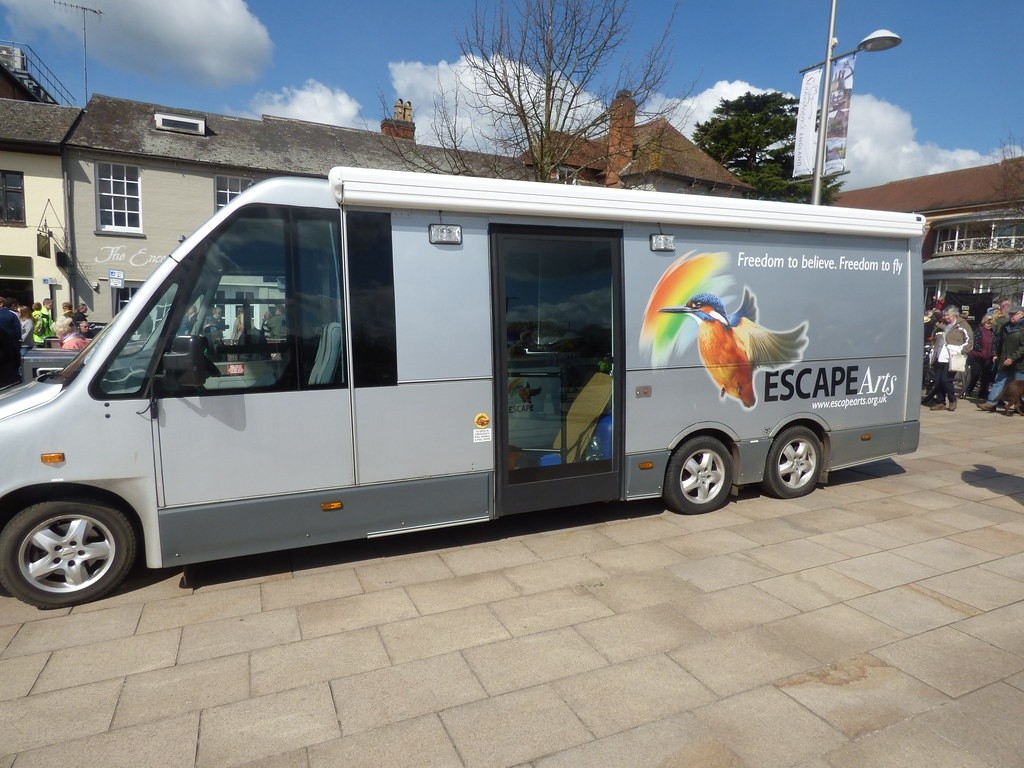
[797,29,903,206]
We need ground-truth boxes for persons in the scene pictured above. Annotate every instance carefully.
[17,306,34,354]
[0,296,74,389]
[138,315,153,338]
[924,296,1024,411]
[233,304,285,339]
[74,303,87,321]
[206,306,224,335]
[53,316,90,351]
[177,305,198,336]
[78,321,90,337]
[961,316,997,399]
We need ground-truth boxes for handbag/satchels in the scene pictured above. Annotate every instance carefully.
[948,354,968,371]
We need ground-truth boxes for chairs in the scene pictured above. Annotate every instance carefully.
[307,321,341,384]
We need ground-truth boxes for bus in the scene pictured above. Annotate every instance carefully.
[0,160,930,612]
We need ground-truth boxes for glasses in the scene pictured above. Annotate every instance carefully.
[83,326,90,328]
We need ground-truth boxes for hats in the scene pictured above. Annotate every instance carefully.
[1008,306,1024,312]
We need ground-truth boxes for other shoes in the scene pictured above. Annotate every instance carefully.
[948,399,957,410]
[959,392,967,399]
[977,403,996,412]
[931,404,946,410]
